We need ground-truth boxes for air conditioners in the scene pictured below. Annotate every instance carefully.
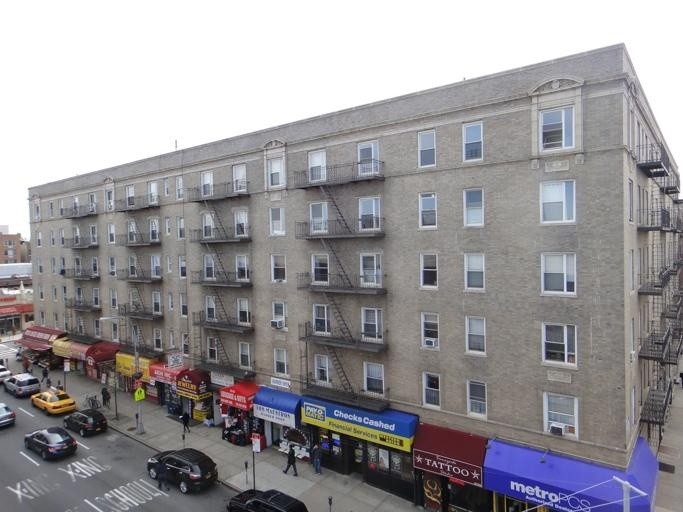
[271,319,283,328]
[550,423,565,436]
[425,338,438,347]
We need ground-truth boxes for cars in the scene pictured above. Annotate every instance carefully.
[24,427,78,458]
[0,402,15,426]
[0,363,12,381]
[64,409,108,437]
[31,390,77,415]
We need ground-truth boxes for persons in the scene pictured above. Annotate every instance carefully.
[180,410,191,434]
[101,386,111,408]
[56,380,64,390]
[22,356,31,373]
[283,444,298,478]
[39,366,50,383]
[152,457,170,491]
[310,441,322,477]
[15,346,22,359]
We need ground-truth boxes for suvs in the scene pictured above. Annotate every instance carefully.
[227,488,309,511]
[147,447,218,494]
[4,374,41,397]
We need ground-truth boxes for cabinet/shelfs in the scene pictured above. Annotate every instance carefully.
[22,350,38,365]
[38,358,50,369]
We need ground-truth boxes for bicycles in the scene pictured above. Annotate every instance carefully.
[43,374,52,388]
[23,366,34,374]
[83,395,101,408]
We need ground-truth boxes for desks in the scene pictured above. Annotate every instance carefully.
[227,428,248,445]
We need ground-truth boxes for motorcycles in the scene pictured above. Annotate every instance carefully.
[16,352,23,361]
